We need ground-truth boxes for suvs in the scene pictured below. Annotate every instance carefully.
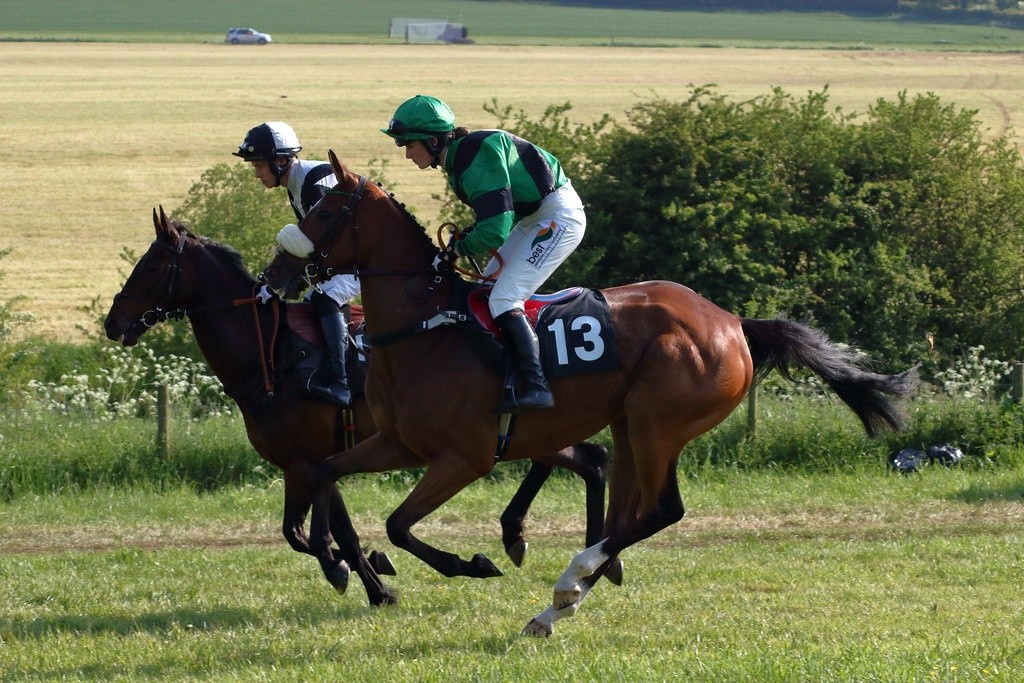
[225,28,271,44]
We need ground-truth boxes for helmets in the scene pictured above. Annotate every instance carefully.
[379,95,457,146]
[232,122,302,161]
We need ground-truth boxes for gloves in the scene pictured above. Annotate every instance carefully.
[432,250,454,274]
[448,233,469,249]
[257,284,276,306]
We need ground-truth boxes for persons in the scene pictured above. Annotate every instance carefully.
[379,95,587,414]
[232,121,363,410]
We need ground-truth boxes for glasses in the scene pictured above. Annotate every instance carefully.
[387,119,449,136]
[239,138,260,156]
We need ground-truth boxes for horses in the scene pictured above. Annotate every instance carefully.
[262,148,922,642]
[103,204,624,609]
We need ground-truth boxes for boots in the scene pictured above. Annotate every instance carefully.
[310,313,352,409]
[492,309,554,408]
